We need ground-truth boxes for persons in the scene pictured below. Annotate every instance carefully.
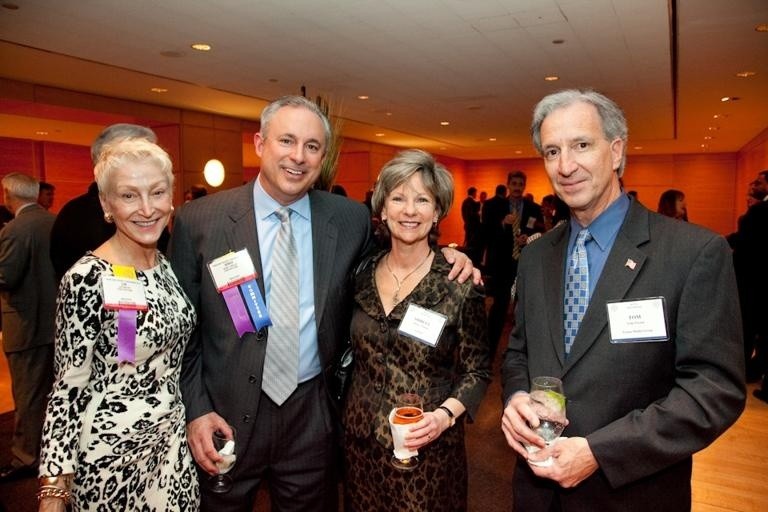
[499,87,747,512]
[342,150,491,511]
[48,122,170,280]
[36,135,204,511]
[168,98,485,511]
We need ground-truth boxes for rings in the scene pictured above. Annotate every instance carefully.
[427,434,432,440]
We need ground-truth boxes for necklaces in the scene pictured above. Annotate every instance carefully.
[385,247,432,306]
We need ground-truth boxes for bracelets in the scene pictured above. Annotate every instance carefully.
[437,242,469,255]
[37,475,70,505]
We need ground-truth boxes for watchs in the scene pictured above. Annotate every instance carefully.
[438,404,456,427]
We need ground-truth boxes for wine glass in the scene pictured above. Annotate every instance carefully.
[203,425,238,494]
[386,393,424,471]
[525,377,570,465]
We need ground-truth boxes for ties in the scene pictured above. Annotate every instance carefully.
[261,207,298,407]
[564,230,591,359]
[512,204,520,260]
